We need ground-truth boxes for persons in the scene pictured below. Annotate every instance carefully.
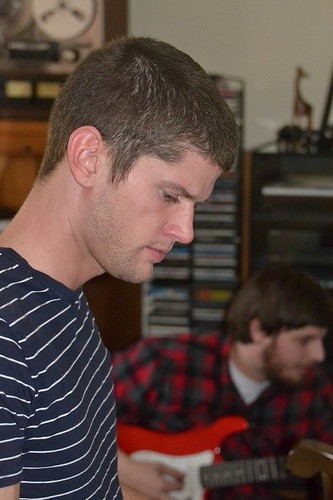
[110,265,333,500]
[0,36,239,500]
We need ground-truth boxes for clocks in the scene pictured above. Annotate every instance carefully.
[34,0,98,39]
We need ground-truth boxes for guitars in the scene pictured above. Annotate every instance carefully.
[116,416,333,500]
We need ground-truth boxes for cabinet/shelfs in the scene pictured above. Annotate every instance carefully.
[142,75,250,341]
